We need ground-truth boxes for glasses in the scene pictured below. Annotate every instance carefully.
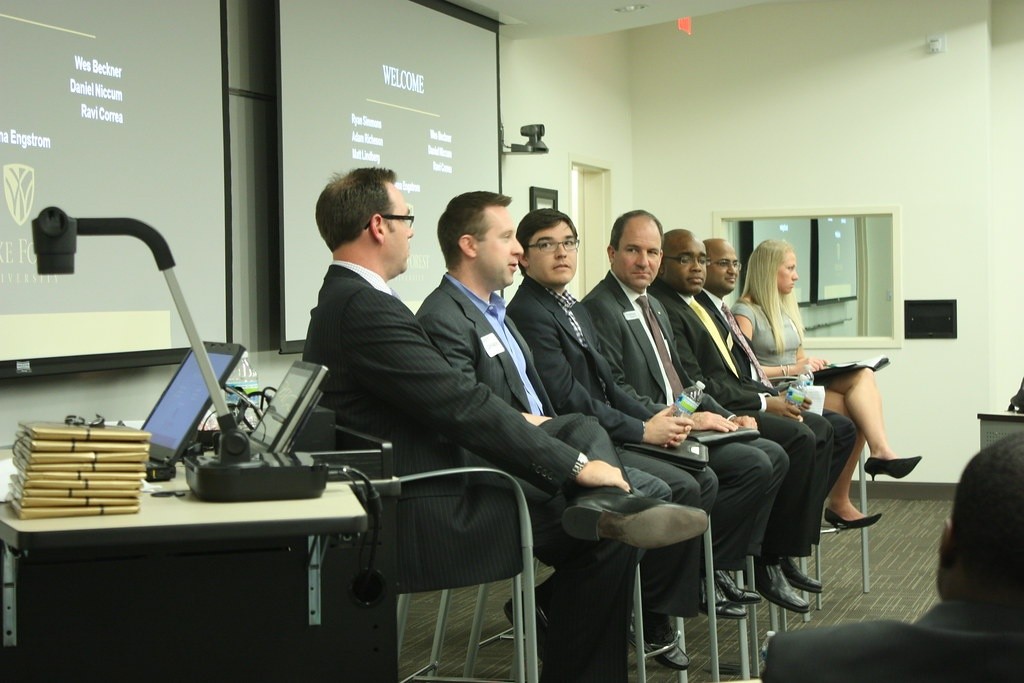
[364,215,414,230]
[712,260,742,271]
[663,256,711,268]
[527,240,580,253]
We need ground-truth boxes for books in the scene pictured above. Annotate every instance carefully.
[8,422,152,520]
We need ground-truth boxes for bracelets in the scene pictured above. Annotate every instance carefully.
[781,364,787,376]
[641,421,645,443]
[787,365,789,376]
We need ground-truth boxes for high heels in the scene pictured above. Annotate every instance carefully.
[824,507,882,535]
[863,456,922,482]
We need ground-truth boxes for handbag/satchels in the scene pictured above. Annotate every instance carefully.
[690,426,761,446]
[622,439,709,472]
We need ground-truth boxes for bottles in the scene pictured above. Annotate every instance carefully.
[761,630,777,671]
[665,381,705,417]
[784,365,814,409]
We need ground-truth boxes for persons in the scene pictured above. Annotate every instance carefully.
[302,169,922,683]
[757,431,1024,683]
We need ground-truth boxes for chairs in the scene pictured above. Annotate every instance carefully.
[337,416,870,683]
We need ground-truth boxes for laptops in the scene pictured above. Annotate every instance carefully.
[141,342,245,482]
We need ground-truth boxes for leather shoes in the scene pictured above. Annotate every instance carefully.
[560,495,710,551]
[697,569,762,618]
[502,589,549,663]
[780,557,824,592]
[743,556,810,615]
[629,612,691,671]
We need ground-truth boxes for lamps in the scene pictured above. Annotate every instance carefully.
[30,208,327,502]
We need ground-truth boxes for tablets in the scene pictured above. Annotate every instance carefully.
[249,359,329,453]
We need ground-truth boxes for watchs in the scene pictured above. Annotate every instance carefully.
[568,452,588,481]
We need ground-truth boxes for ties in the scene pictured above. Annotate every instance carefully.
[689,297,739,379]
[636,295,684,403]
[721,302,774,389]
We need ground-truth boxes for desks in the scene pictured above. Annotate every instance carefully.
[1,425,398,683]
